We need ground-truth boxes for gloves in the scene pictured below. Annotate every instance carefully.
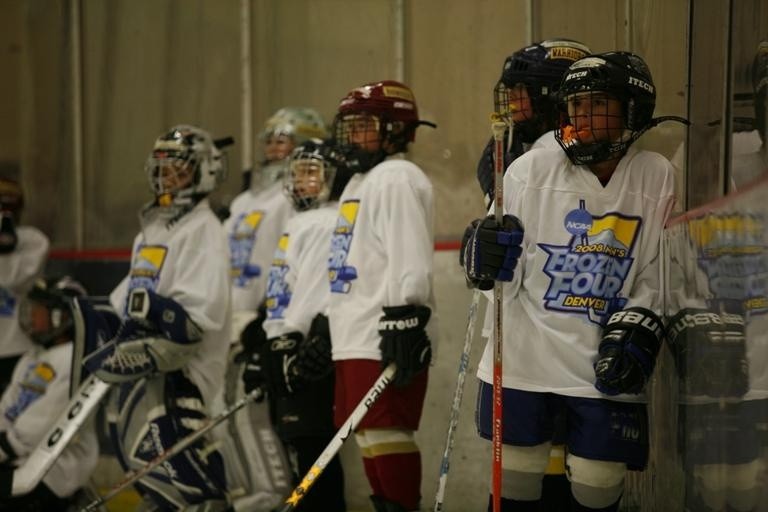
[592,306,668,395]
[670,308,750,397]
[240,318,334,400]
[458,214,526,291]
[377,304,432,387]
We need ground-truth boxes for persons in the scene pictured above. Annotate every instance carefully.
[464,51,674,511]
[110,126,235,512]
[327,81,432,511]
[0,180,99,512]
[221,107,348,511]
[461,41,591,511]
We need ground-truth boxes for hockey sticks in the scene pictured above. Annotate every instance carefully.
[0,371,111,496]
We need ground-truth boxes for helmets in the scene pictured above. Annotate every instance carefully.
[257,104,349,211]
[331,79,420,173]
[144,123,224,205]
[17,274,87,350]
[549,48,655,166]
[488,35,591,133]
